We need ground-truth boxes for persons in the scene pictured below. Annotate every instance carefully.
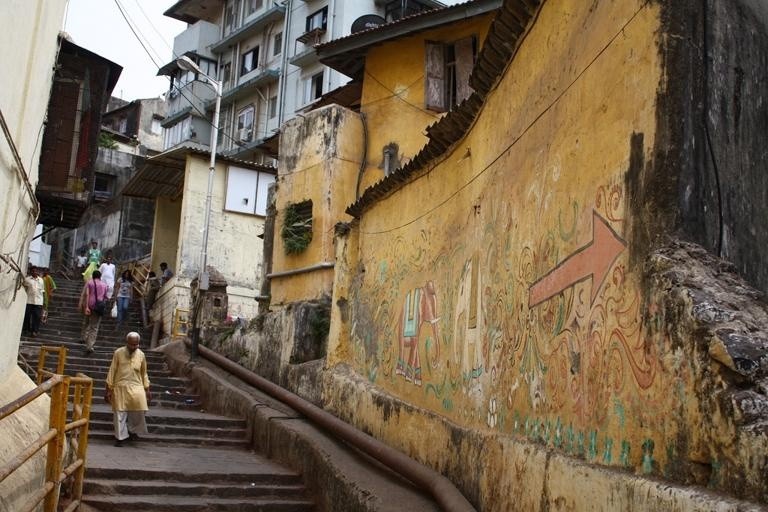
[115,270,134,330]
[22,265,57,337]
[160,262,173,288]
[104,331,152,446]
[144,271,161,310]
[74,241,116,353]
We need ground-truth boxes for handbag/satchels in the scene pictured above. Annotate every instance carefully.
[94,301,106,314]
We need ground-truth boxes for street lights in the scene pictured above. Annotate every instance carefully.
[177,55,223,290]
